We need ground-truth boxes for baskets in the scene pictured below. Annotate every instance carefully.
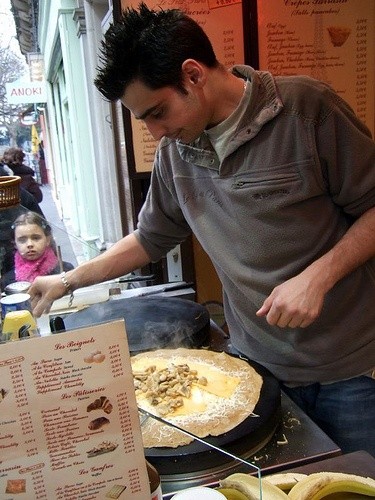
[0,176,21,209]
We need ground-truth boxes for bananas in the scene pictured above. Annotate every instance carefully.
[211,471,375,500]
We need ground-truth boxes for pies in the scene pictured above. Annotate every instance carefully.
[130,348,263,448]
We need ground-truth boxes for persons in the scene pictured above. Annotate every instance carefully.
[0,148,75,293]
[25,0,375,456]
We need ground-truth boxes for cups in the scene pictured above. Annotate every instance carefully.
[0,293,32,319]
[2,310,36,339]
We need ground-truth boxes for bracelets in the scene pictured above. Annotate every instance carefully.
[61,271,75,308]
